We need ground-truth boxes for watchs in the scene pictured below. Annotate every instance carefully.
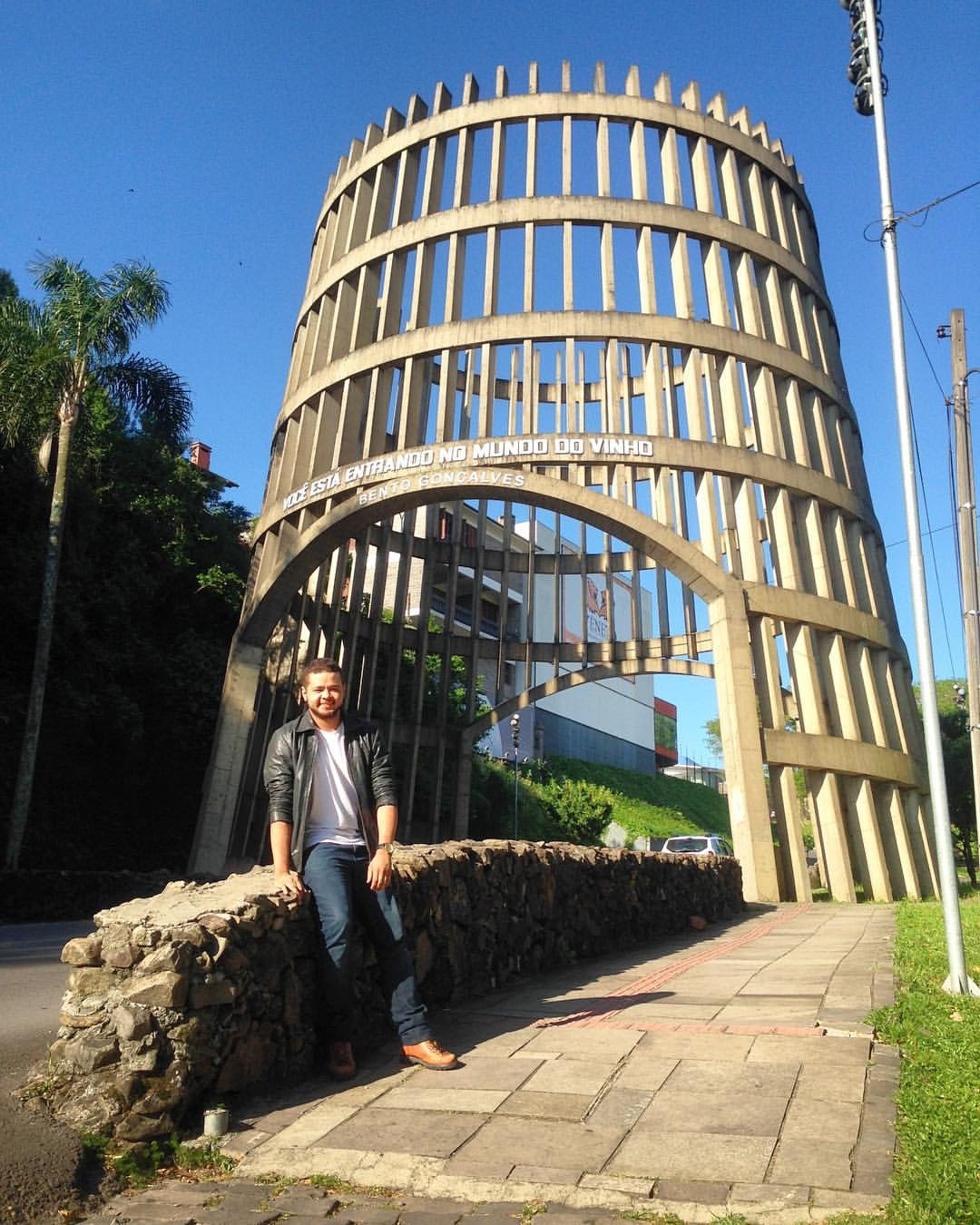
[376,842,394,854]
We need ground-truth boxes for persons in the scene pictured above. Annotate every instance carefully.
[264,657,457,1079]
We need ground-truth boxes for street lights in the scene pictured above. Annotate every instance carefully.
[508,712,522,842]
[840,0,980,1000]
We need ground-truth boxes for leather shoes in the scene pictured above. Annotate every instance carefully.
[319,1037,355,1080]
[400,1037,458,1071]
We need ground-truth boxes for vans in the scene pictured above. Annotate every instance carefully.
[660,834,734,857]
